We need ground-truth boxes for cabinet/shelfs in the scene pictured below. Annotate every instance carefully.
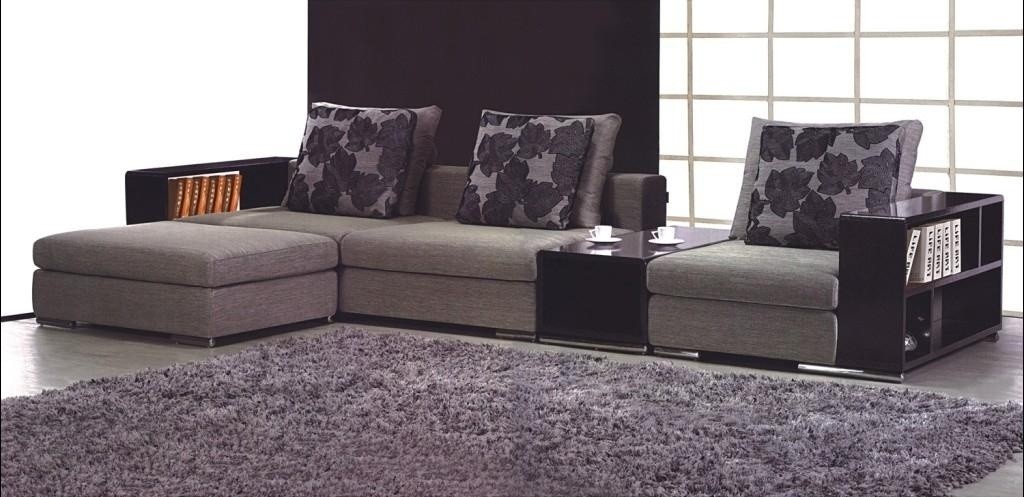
[838,192,1004,373]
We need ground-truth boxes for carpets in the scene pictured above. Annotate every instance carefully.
[1,324,1023,497]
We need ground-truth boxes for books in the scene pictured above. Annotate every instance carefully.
[906,218,961,288]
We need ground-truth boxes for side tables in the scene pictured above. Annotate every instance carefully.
[535,225,730,360]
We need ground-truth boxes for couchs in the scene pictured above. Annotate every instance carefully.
[32,158,668,347]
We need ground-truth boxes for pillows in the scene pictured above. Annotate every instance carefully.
[455,112,595,231]
[744,125,900,251]
[728,117,922,240]
[280,101,442,217]
[480,109,621,228]
[286,106,417,218]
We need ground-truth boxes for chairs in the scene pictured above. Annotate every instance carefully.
[647,189,931,365]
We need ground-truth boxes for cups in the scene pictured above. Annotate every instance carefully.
[588,226,613,241]
[649,225,677,241]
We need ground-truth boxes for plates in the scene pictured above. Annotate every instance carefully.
[647,236,685,246]
[587,236,624,243]
